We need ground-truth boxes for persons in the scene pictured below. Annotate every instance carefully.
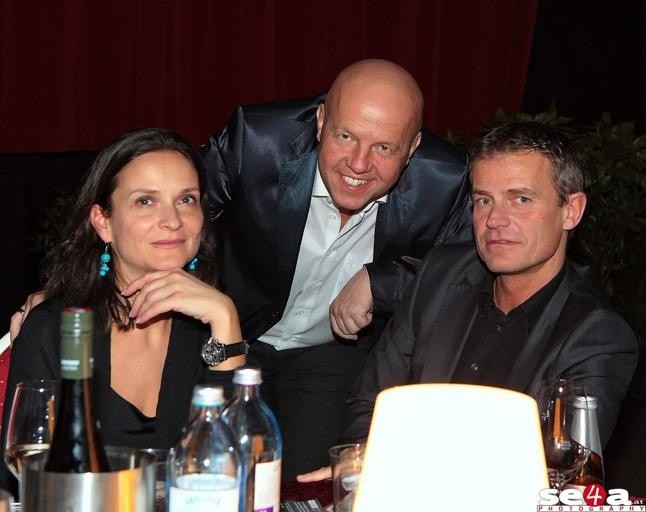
[0,59,639,512]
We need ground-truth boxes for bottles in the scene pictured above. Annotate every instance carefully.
[567,394,605,511]
[44,306,112,471]
[163,369,283,512]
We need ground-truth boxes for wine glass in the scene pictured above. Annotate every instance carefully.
[4,381,58,506]
[534,380,591,512]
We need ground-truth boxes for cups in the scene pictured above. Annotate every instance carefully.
[329,444,364,512]
